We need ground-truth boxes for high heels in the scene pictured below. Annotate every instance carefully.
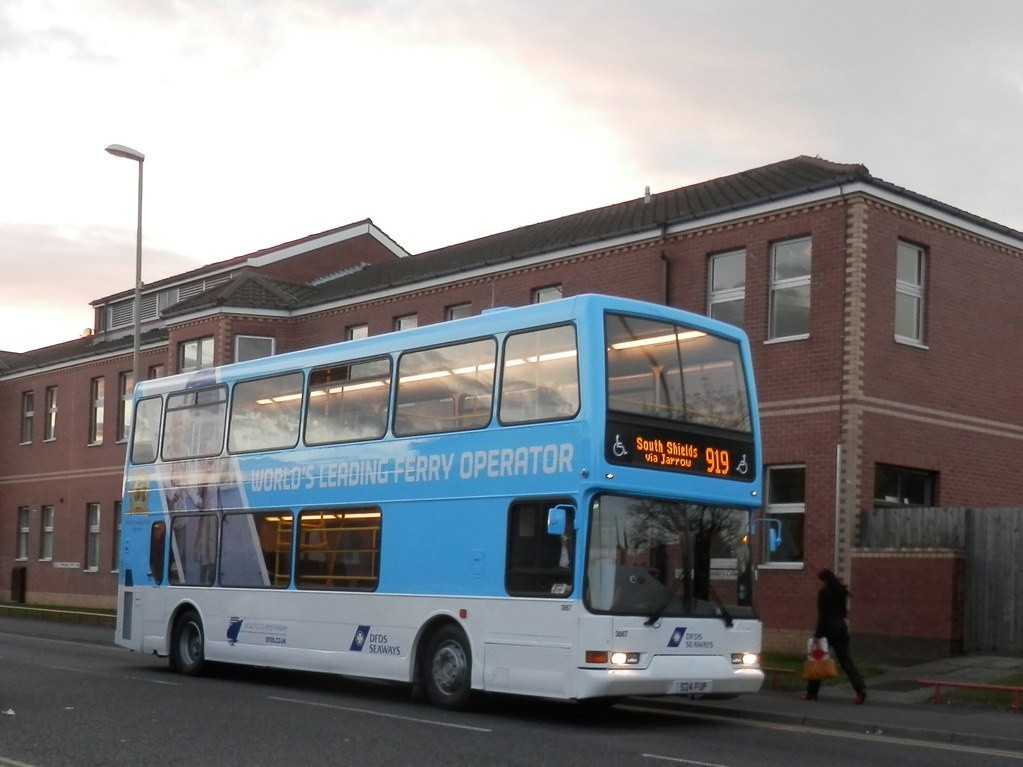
[855,691,866,705]
[800,693,817,700]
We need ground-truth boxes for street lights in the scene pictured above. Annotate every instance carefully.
[105,143,145,396]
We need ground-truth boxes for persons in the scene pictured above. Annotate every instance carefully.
[197,406,230,587]
[799,565,870,704]
[159,403,206,584]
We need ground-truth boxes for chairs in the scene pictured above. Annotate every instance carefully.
[235,388,573,586]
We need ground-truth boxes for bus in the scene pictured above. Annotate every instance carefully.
[115,291,768,716]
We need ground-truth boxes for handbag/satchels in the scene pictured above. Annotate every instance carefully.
[805,637,829,662]
[802,654,839,679]
